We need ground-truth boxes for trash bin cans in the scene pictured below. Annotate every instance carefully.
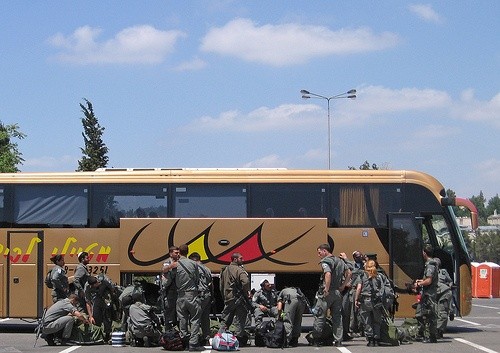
[476,262,500,298]
[470,261,479,297]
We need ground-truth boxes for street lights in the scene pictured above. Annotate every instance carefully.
[301,87,357,169]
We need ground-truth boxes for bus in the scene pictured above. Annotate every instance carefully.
[0,168,477,336]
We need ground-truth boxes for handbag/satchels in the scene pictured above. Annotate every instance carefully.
[70,323,101,342]
[305,319,334,346]
[370,296,382,310]
[45,270,54,289]
[400,318,418,341]
[386,323,400,346]
[159,328,188,351]
[101,321,124,345]
[200,320,285,351]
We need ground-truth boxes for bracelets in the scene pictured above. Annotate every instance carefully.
[417,283,420,287]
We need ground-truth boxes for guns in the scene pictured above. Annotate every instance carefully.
[296,288,317,321]
[33,308,47,348]
[371,293,391,311]
[414,300,438,320]
[284,294,291,304]
[221,282,255,319]
[158,275,167,328]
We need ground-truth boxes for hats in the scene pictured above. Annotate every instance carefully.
[366,253,377,259]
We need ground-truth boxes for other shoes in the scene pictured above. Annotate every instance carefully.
[237,338,245,347]
[56,340,66,346]
[335,342,342,346]
[189,344,205,351]
[367,341,374,347]
[450,314,454,321]
[130,339,136,347]
[374,340,379,346]
[343,332,362,341]
[143,336,148,347]
[416,329,443,343]
[40,333,56,346]
[282,342,298,348]
[312,342,320,346]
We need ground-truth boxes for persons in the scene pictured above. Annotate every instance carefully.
[162,245,212,351]
[84,274,114,346]
[277,286,305,348]
[339,250,396,347]
[50,255,71,305]
[220,253,251,347]
[118,282,162,347]
[307,244,352,347]
[414,244,453,343]
[251,280,279,327]
[42,294,90,346]
[74,252,91,319]
